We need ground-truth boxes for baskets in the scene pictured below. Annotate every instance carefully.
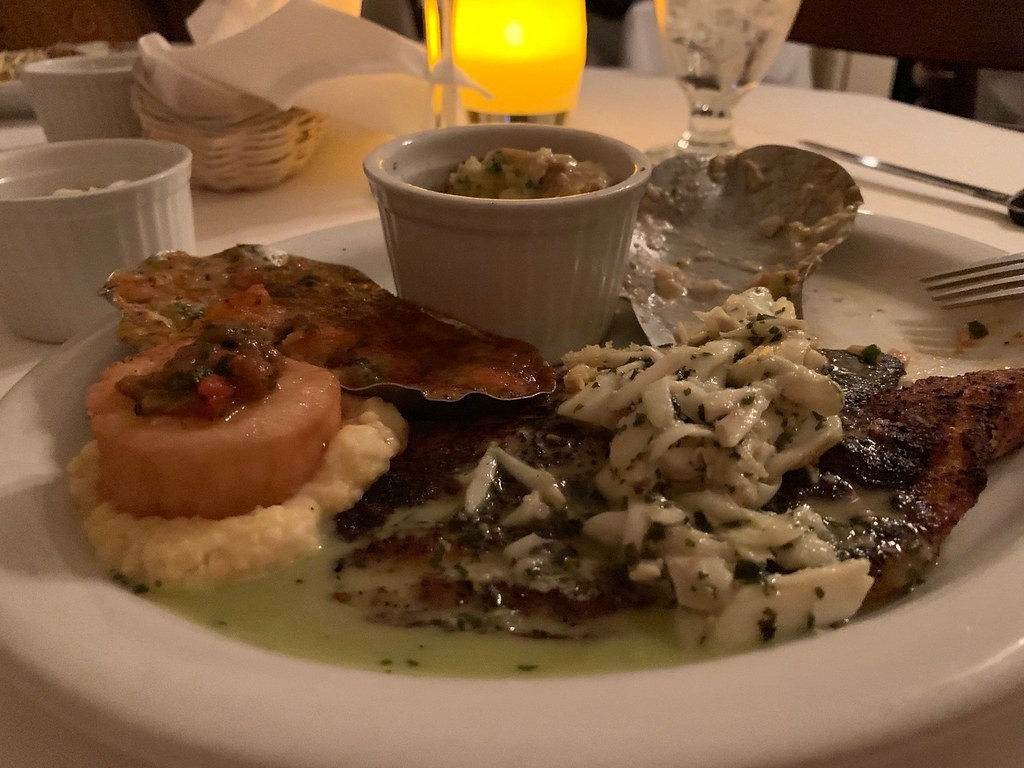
[129,103,328,195]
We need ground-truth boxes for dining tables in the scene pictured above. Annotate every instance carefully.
[1,36,1024,768]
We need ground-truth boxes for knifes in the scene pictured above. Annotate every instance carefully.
[804,138,1024,222]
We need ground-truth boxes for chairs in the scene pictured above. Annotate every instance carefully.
[789,0,1023,118]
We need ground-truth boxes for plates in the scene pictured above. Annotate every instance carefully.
[0,173,1024,768]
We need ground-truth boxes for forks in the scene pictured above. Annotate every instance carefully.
[918,249,1023,311]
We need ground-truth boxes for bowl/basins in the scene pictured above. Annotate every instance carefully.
[20,45,150,147]
[1,132,199,347]
[365,123,659,372]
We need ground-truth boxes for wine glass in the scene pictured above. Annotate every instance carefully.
[642,0,799,177]
